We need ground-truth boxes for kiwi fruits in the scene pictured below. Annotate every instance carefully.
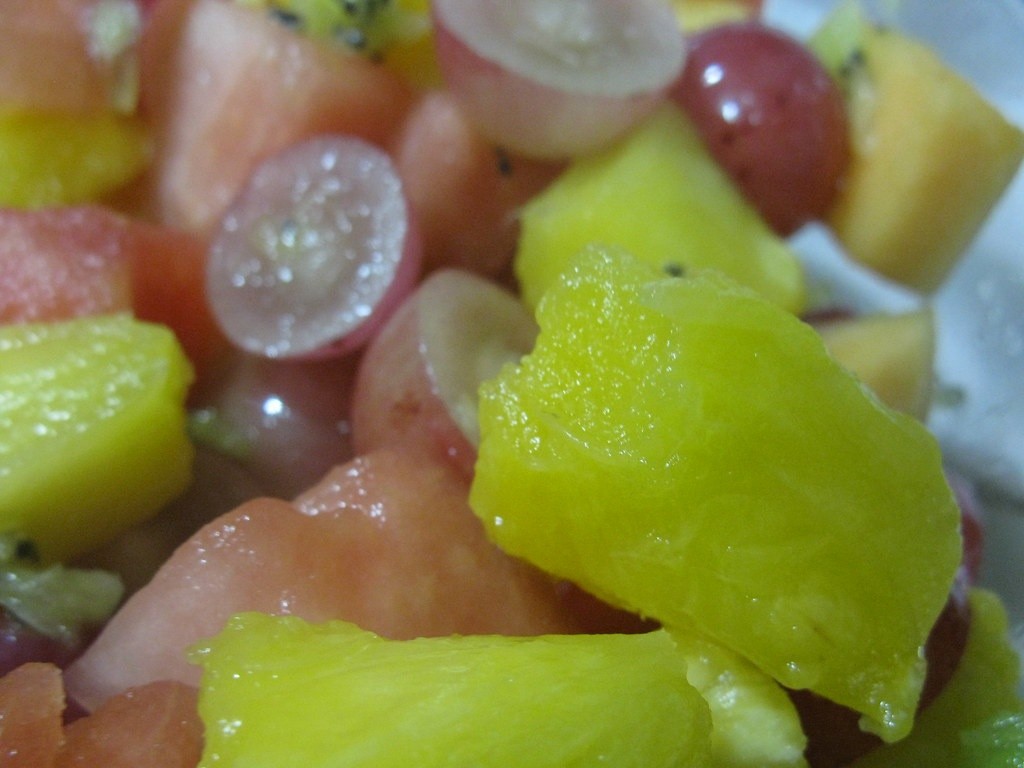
[0,0,1024,768]
